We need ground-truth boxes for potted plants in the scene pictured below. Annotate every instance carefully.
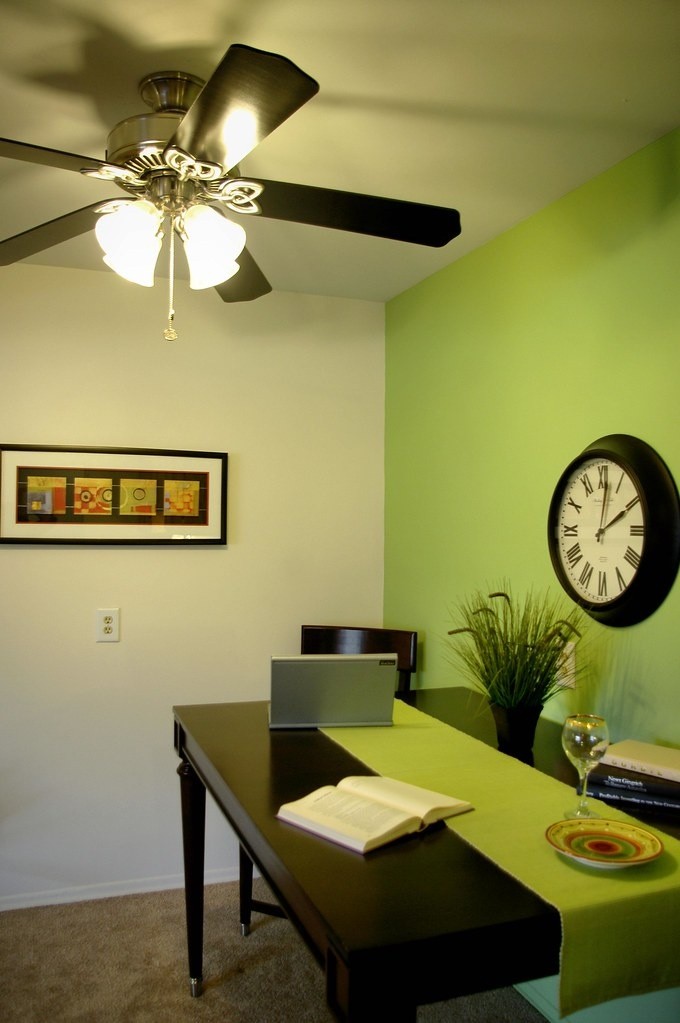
[438,577,606,752]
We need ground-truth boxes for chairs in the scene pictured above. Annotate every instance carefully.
[237,624,419,936]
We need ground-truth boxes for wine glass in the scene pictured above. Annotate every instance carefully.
[562,714,609,820]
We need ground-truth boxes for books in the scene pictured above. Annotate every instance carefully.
[577,739,680,809]
[274,776,472,855]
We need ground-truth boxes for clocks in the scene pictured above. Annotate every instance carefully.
[547,434,680,629]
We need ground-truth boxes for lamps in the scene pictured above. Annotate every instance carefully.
[97,199,246,290]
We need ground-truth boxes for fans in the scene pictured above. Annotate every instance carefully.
[0,44,463,303]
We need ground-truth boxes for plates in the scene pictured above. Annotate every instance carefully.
[546,819,663,869]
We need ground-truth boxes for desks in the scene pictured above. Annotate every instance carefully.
[172,685,680,1023]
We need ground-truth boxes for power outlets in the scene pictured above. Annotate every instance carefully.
[552,643,576,689]
[96,608,120,642]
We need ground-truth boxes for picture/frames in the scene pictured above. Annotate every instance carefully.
[0,443,228,544]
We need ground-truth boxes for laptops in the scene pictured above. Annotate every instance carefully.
[264,652,399,729]
